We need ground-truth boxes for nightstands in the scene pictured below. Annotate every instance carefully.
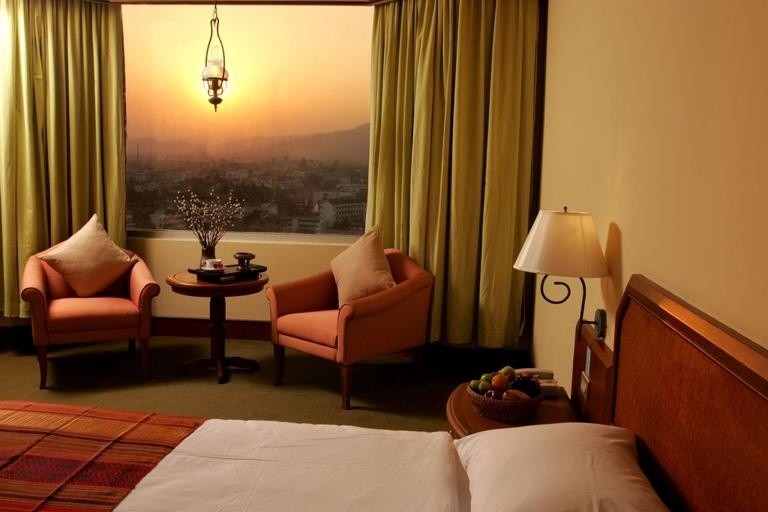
[446,382,585,440]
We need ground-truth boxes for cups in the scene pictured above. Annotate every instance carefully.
[206,259,222,268]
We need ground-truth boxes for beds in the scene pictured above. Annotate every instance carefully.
[0,274,767,512]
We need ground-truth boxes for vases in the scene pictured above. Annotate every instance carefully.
[198,246,220,269]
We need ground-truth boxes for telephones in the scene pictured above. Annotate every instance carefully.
[515,368,559,398]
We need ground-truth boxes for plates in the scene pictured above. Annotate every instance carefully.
[201,265,225,272]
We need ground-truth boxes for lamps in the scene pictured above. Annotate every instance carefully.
[513,205,612,342]
[200,0,230,112]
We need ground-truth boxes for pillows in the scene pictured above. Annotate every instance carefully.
[40,213,140,298]
[452,421,670,512]
[329,224,398,309]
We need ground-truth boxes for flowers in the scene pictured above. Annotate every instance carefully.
[174,186,246,246]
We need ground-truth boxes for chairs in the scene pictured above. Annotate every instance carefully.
[265,248,434,410]
[21,245,159,391]
